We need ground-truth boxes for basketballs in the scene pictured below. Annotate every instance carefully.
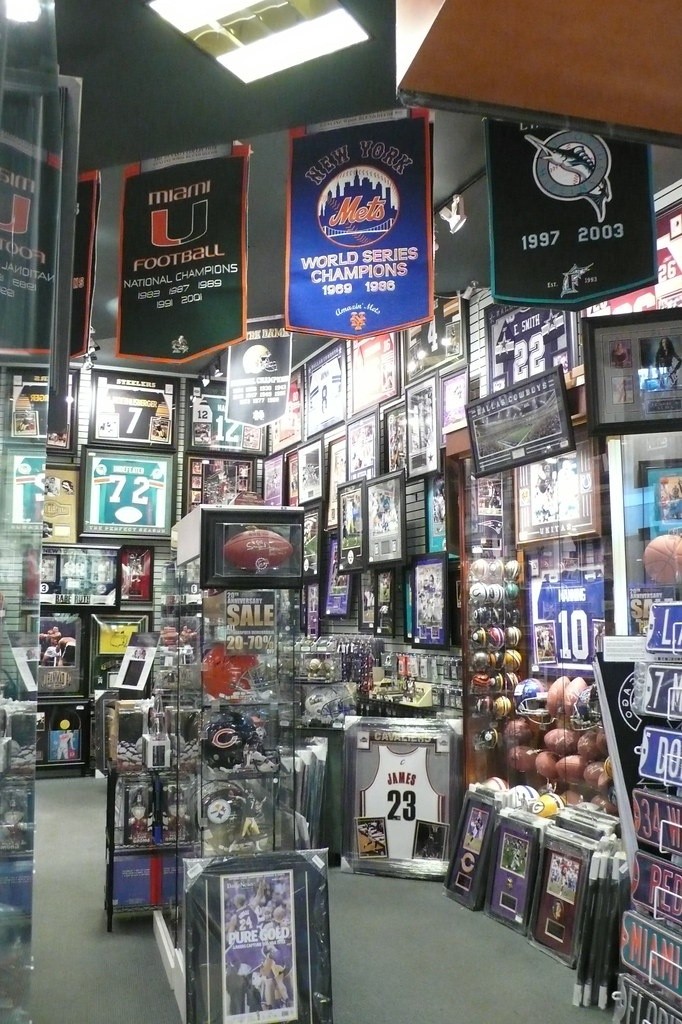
[644,534,682,585]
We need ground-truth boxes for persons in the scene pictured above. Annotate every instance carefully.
[224,872,294,1016]
[609,335,681,405]
[13,410,261,506]
[465,809,579,900]
[331,419,585,664]
[39,625,198,759]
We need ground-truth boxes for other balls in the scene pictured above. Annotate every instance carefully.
[481,777,566,821]
[469,554,521,748]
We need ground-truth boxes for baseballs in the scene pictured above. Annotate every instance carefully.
[323,660,338,677]
[307,658,322,674]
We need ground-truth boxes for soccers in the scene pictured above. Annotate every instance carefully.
[199,713,262,772]
[195,789,259,854]
[201,647,259,702]
[305,687,341,720]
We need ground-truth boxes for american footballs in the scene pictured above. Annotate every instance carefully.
[506,675,619,813]
[222,529,292,569]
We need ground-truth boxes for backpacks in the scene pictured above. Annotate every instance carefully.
[192,782,262,851]
[203,709,263,771]
